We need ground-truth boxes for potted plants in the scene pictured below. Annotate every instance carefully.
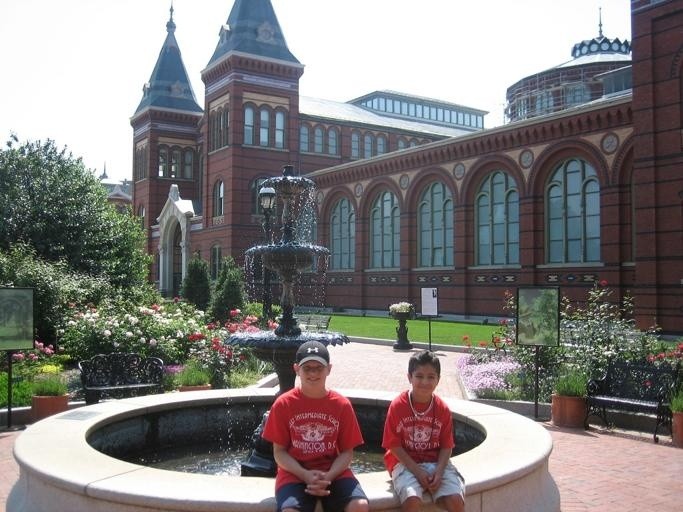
[31,374,68,422]
[550,365,589,429]
[179,359,213,394]
[668,387,683,446]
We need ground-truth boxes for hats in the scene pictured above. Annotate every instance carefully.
[295,339,330,367]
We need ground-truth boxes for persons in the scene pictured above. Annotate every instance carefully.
[382,349,466,511]
[261,339,369,512]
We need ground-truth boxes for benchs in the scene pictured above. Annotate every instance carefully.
[294,315,331,332]
[586,362,678,443]
[79,353,167,405]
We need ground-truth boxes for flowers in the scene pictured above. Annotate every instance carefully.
[388,302,416,313]
